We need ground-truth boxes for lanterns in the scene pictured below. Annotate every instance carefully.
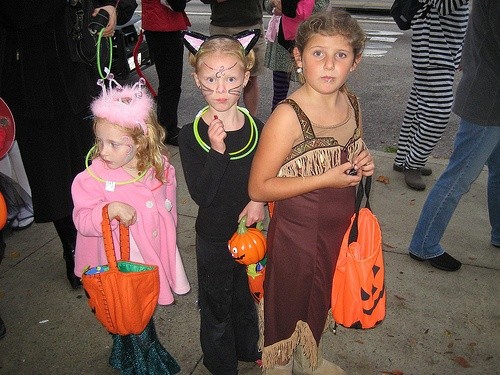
[228,227,267,266]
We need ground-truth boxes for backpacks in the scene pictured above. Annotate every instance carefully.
[389,0,423,31]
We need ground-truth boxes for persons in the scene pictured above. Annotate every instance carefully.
[392,0,472,191]
[0,0,138,289]
[178,28,266,375]
[200,0,263,116]
[248,10,375,375]
[141,0,191,145]
[70,73,191,375]
[408,0,500,272]
[263,0,332,110]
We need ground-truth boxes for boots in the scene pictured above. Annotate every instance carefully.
[262,356,293,375]
[293,346,347,375]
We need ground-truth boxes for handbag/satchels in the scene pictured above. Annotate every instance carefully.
[331,175,385,329]
[81,203,160,335]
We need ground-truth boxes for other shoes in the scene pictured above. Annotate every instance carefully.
[62,250,82,288]
[404,168,426,191]
[393,163,432,176]
[409,252,461,272]
[161,127,181,147]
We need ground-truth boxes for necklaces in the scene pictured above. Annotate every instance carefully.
[193,105,259,160]
[85,144,147,185]
